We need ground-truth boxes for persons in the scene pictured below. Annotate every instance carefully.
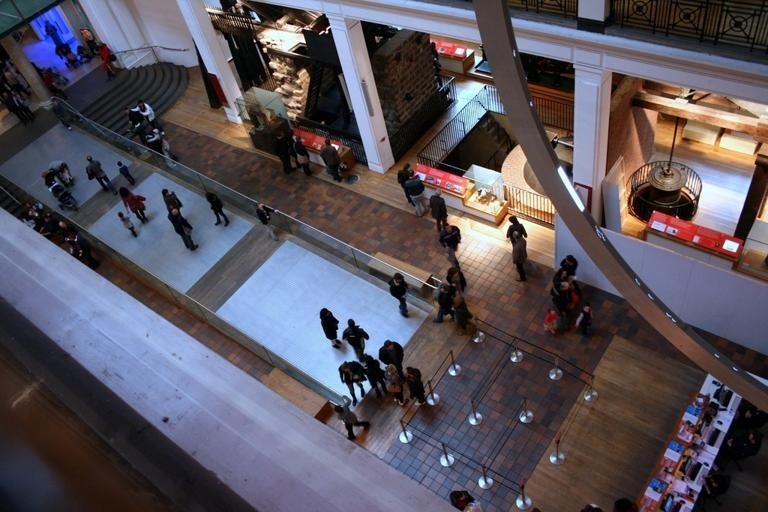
[2,90,29,126]
[398,163,429,216]
[43,20,116,76]
[390,273,409,317]
[318,140,343,183]
[273,127,311,177]
[253,200,280,239]
[10,91,36,120]
[543,254,595,339]
[433,267,473,336]
[447,487,476,511]
[321,308,369,362]
[338,340,426,406]
[335,396,370,440]
[429,189,462,270]
[500,213,530,281]
[19,99,230,269]
[39,73,71,98]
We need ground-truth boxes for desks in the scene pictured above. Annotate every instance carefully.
[635,372,768,512]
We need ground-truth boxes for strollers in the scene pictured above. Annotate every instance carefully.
[50,182,78,212]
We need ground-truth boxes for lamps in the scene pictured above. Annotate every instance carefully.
[626,115,703,222]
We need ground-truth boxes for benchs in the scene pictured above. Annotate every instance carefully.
[262,367,333,421]
[368,253,433,297]
